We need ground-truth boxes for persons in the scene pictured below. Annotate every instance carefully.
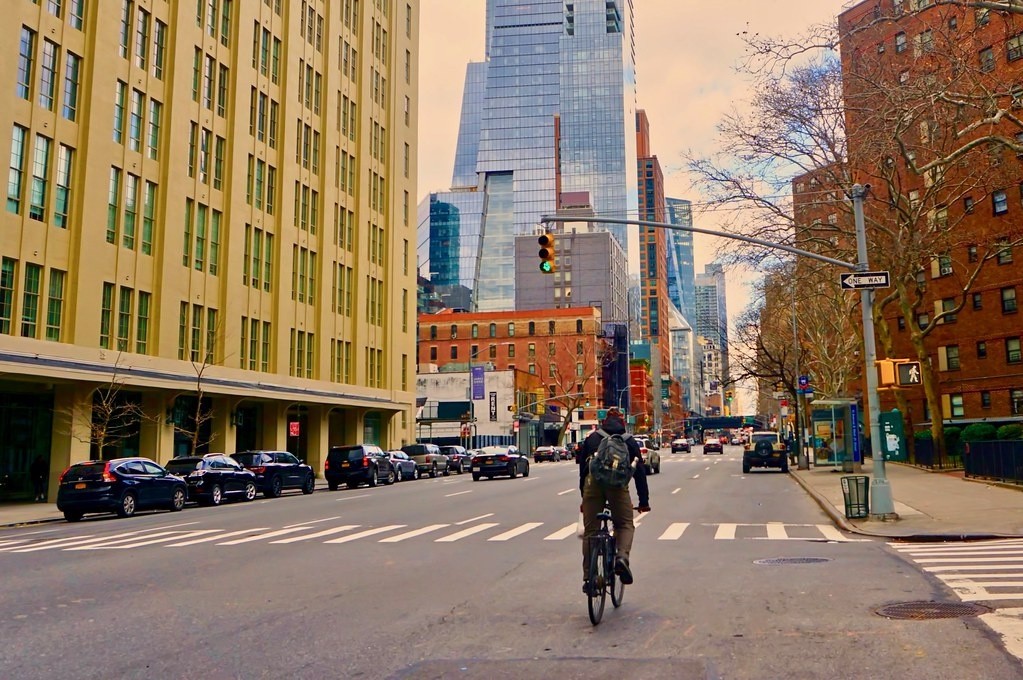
[575,407,650,593]
[858,427,866,465]
[30,454,49,502]
[821,438,832,450]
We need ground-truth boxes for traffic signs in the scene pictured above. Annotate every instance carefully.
[840,272,890,290]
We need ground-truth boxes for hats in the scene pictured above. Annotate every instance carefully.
[603,407,626,427]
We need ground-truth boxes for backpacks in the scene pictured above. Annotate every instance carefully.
[586,428,637,487]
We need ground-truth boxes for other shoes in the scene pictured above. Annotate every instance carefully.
[615,556,633,584]
[583,581,590,592]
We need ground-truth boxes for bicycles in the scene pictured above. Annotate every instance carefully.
[580,503,652,625]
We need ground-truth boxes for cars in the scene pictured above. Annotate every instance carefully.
[534,440,579,463]
[57,457,190,522]
[731,439,740,446]
[703,439,723,454]
[438,445,473,474]
[632,435,660,475]
[386,451,419,481]
[671,439,692,453]
[471,444,529,481]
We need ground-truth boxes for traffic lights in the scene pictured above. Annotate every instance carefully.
[725,391,733,400]
[644,415,649,423]
[586,396,590,407]
[895,361,923,386]
[538,234,555,274]
[687,421,690,427]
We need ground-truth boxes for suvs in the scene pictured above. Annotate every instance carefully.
[742,431,788,473]
[401,443,450,478]
[164,452,258,506]
[230,452,315,498]
[325,444,397,490]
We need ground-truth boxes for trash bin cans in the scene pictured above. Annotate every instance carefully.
[840,476,870,518]
